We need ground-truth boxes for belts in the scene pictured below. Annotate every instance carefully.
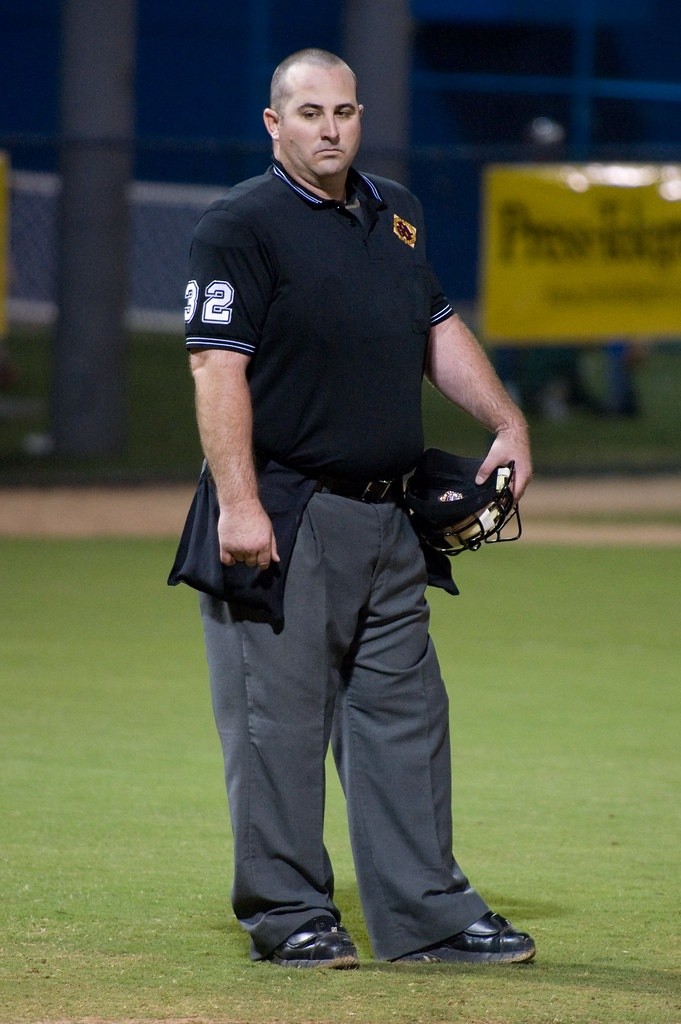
[316,476,402,505]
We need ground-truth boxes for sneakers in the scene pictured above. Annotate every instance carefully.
[394,909,536,962]
[270,916,359,972]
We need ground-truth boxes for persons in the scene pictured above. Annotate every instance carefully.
[164,44,540,972]
